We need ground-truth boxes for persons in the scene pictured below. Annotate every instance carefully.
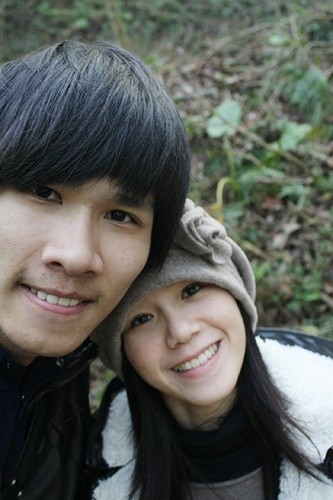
[90,197,332,500]
[0,41,192,499]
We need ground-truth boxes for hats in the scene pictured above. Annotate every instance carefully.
[88,196,258,383]
